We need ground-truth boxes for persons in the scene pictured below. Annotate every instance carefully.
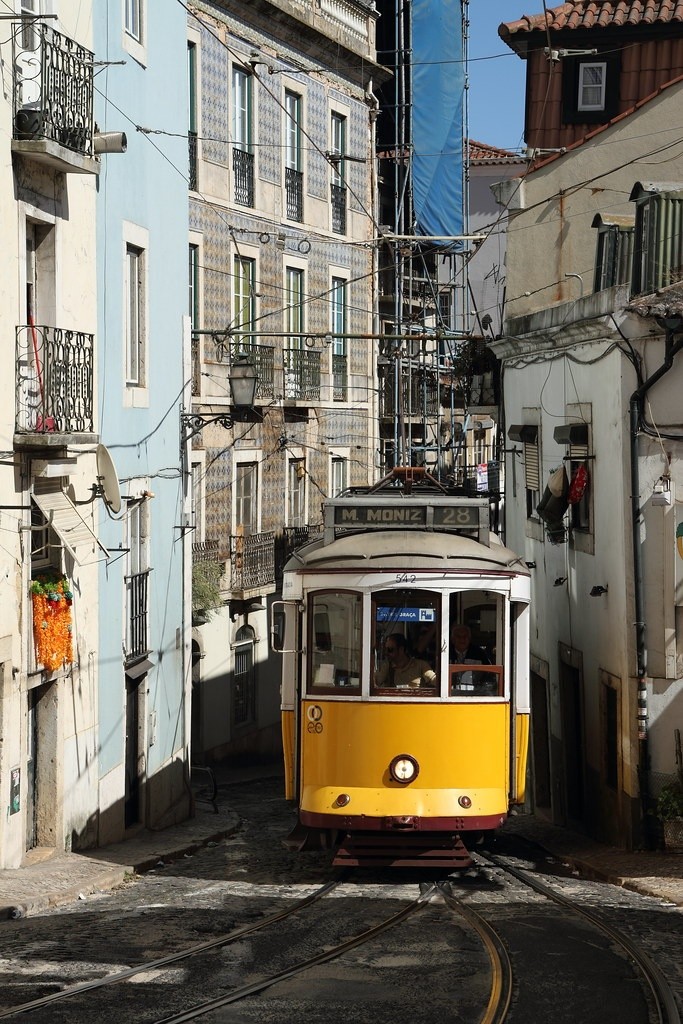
[375,634,438,690]
[417,621,500,689]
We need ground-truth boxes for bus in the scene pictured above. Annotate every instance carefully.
[268,469,536,871]
